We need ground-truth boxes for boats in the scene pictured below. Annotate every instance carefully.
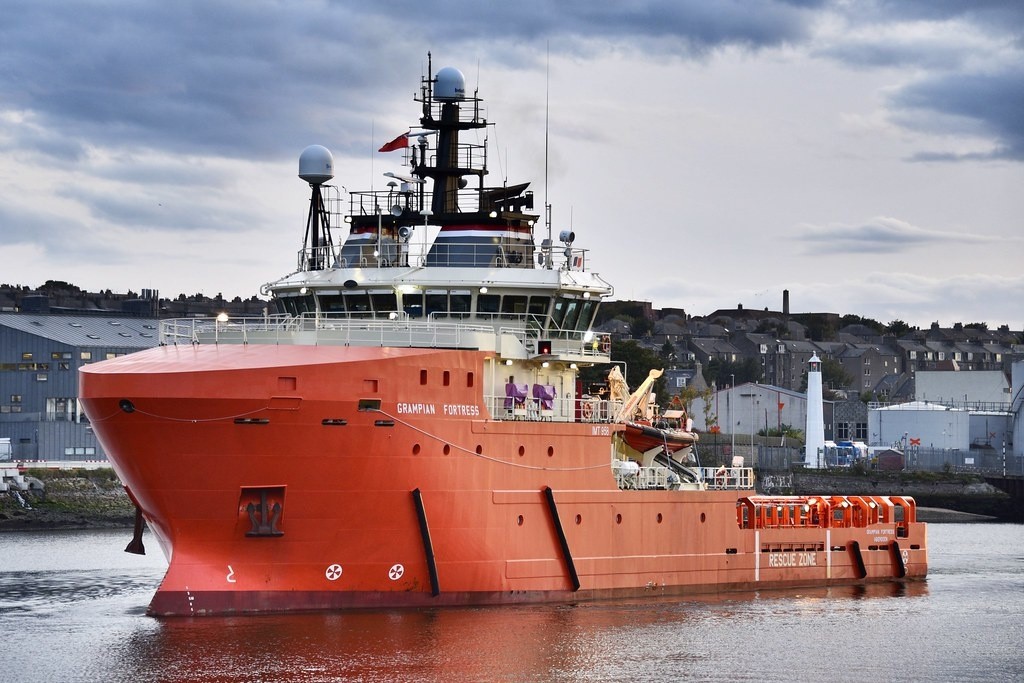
[615,418,699,456]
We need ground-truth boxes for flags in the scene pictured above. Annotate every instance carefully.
[377,131,409,152]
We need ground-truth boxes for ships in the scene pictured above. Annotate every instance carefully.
[76,47,931,622]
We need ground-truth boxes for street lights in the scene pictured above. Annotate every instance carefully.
[730,373,735,468]
